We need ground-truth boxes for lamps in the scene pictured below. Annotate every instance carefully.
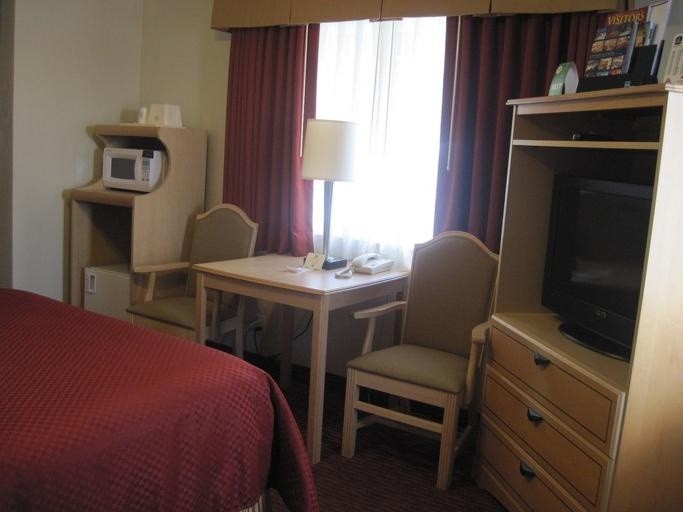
[298,117,357,268]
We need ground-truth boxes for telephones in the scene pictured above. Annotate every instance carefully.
[351,254,394,275]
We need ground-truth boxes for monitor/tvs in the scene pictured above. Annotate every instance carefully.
[539,171,654,362]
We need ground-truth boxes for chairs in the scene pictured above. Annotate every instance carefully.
[340,230,500,489]
[126,201,258,359]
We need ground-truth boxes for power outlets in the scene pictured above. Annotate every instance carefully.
[255,313,265,336]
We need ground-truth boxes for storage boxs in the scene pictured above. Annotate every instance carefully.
[82,267,131,321]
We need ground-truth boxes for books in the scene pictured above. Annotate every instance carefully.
[585,4,655,77]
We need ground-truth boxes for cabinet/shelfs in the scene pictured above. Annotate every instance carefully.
[61,124,208,321]
[465,85,683,512]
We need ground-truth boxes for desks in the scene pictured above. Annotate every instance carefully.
[193,251,410,466]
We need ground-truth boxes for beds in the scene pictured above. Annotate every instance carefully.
[0,285,272,509]
